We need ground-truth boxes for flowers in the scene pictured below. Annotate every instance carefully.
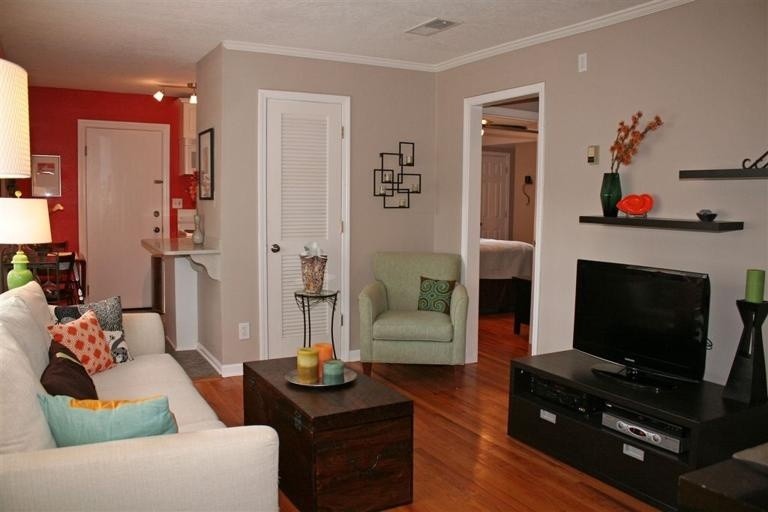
[606,110,664,171]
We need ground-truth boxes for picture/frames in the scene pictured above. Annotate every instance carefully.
[198,127,215,200]
[30,154,63,198]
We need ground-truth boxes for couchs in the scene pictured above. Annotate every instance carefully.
[0,280,280,512]
[357,249,468,369]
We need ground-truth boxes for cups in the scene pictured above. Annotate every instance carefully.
[323,359,345,385]
[299,255,328,294]
[314,341,333,377]
[295,348,318,384]
[745,268,765,303]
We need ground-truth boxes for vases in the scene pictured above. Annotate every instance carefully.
[599,172,622,216]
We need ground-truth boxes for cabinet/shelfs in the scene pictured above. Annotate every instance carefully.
[1,252,88,305]
[506,350,768,512]
[179,100,197,175]
[577,167,767,234]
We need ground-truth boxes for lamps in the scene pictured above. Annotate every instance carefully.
[0,190,53,290]
[0,58,33,179]
[153,81,199,106]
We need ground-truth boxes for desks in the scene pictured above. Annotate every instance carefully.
[293,290,339,360]
[479,237,534,335]
[142,236,220,355]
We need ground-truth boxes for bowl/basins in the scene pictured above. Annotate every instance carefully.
[696,212,718,223]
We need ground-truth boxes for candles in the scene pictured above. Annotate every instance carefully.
[744,270,764,302]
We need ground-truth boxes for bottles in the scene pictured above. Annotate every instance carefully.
[718,299,768,404]
[191,215,204,245]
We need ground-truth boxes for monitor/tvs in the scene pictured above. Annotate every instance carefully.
[572,258,710,390]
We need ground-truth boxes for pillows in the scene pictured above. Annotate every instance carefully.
[36,391,179,449]
[53,296,134,367]
[40,338,99,401]
[46,309,116,376]
[418,275,457,315]
